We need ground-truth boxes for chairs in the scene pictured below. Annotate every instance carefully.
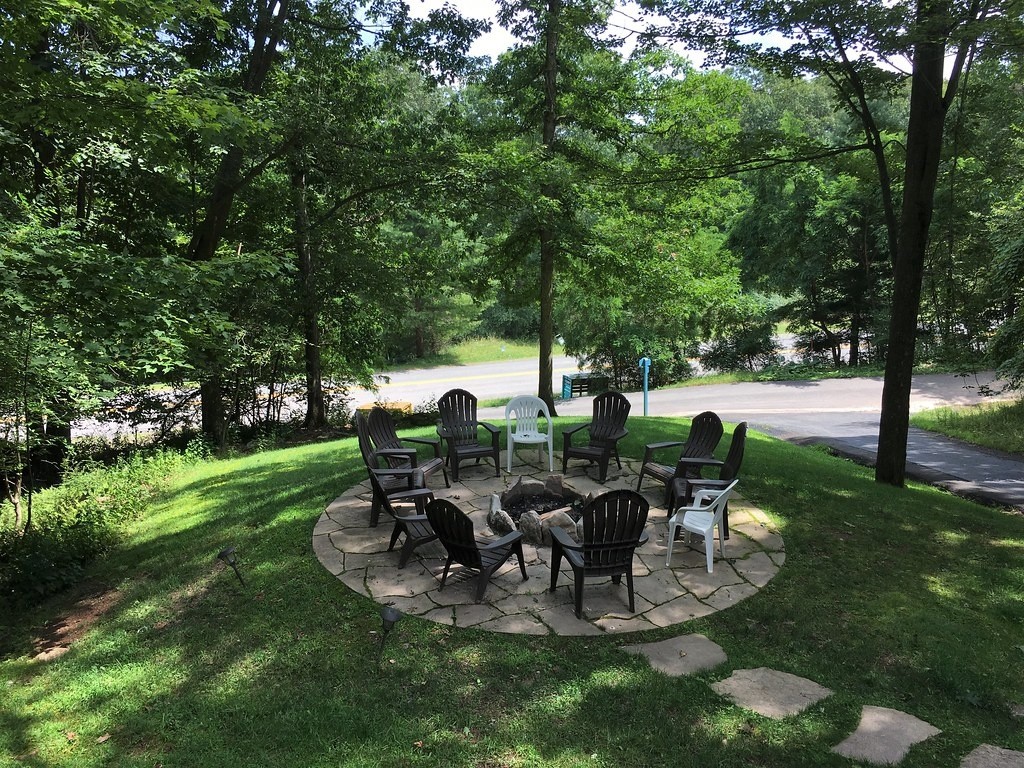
[355,411,428,528]
[667,422,748,540]
[367,407,451,502]
[665,478,740,574]
[562,391,631,484]
[367,466,439,569]
[637,411,724,509]
[436,389,501,482]
[424,499,529,605]
[549,489,650,619]
[505,395,553,473]
